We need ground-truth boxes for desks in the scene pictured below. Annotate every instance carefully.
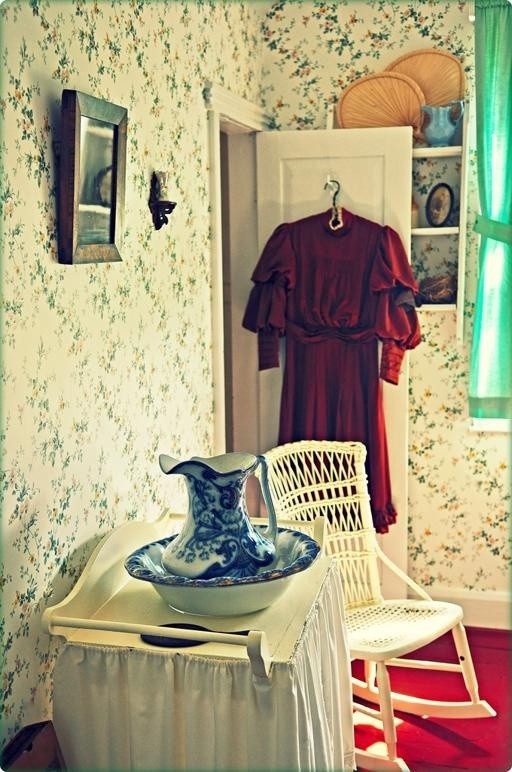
[39,515,358,772]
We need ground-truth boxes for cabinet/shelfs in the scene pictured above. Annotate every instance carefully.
[322,94,474,347]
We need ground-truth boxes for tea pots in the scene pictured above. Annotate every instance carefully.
[424,100,463,148]
[158,454,274,578]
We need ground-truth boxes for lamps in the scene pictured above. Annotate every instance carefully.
[146,169,177,229]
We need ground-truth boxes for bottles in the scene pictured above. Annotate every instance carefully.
[412,188,420,227]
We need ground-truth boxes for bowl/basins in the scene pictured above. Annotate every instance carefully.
[123,523,321,616]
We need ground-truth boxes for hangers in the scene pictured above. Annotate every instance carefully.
[277,175,399,244]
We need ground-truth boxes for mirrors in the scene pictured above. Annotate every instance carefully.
[56,85,130,265]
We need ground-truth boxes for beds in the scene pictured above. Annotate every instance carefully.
[251,440,500,772]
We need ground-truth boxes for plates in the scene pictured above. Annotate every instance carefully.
[425,183,455,227]
